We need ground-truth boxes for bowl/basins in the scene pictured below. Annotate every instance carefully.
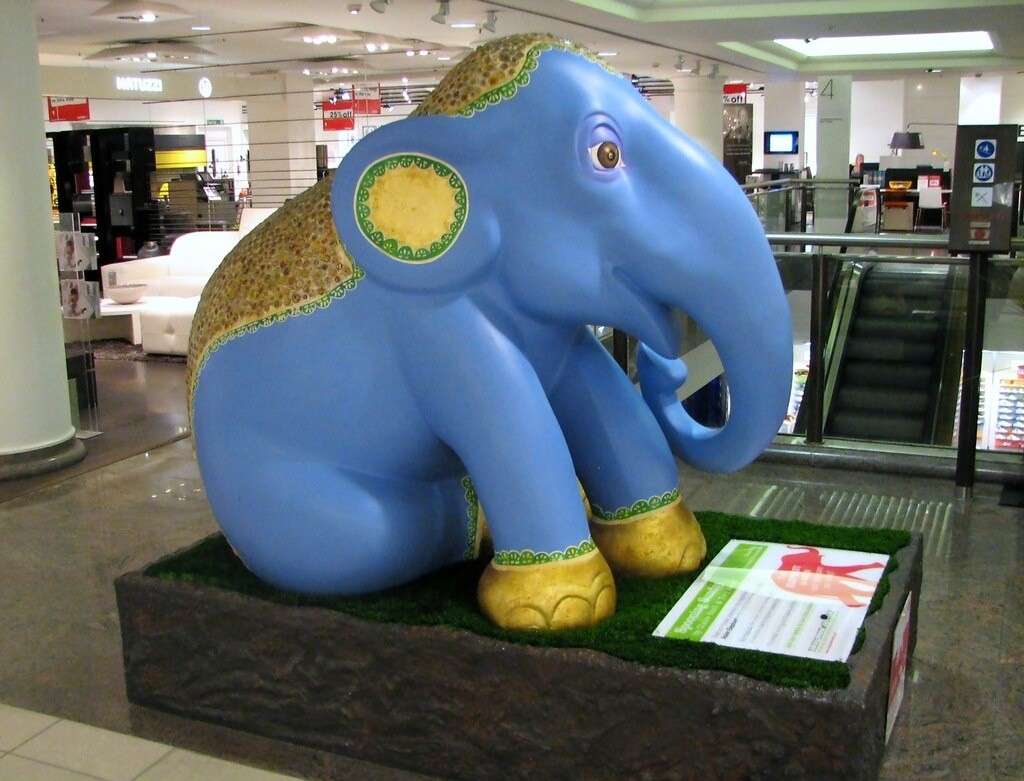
[888,182,912,188]
[105,284,148,303]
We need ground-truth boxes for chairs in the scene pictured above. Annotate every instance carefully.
[914,187,947,231]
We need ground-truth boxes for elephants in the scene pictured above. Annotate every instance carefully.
[186,31,794,629]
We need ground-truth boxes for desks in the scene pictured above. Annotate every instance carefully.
[879,189,952,225]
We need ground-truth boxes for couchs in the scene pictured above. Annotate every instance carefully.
[100,231,241,297]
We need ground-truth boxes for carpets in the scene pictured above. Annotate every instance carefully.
[64,340,187,363]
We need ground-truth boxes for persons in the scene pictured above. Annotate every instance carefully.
[851,153,865,178]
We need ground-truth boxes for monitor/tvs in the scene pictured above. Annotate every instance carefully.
[765,131,798,155]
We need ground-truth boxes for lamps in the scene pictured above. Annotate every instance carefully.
[890,122,958,148]
[672,53,719,80]
[431,0,450,24]
[482,9,497,32]
[370,0,388,13]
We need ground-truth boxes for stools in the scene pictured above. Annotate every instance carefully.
[140,296,194,355]
